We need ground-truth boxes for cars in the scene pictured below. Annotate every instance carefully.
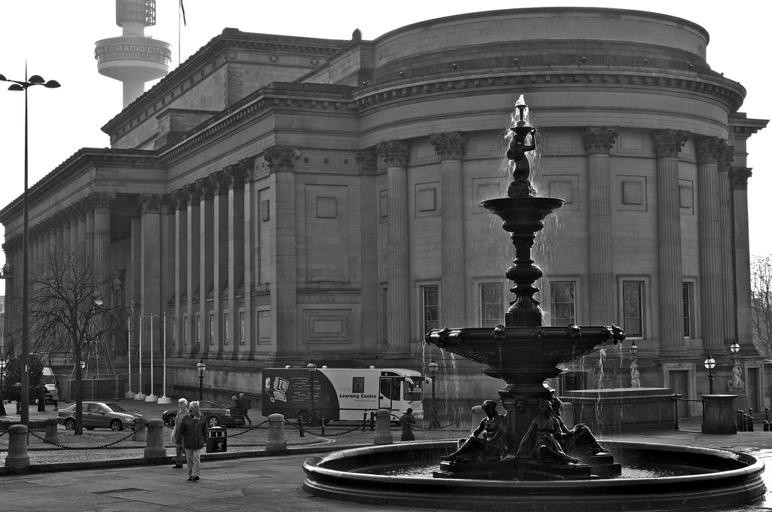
[162,399,246,428]
[57,400,145,432]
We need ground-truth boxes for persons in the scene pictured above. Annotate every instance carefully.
[552,397,608,452]
[175,401,209,481]
[232,396,244,417]
[439,399,507,463]
[629,360,641,387]
[399,407,416,441]
[6,382,61,415]
[239,392,252,426]
[170,397,187,469]
[506,131,536,180]
[516,399,581,463]
[732,363,744,386]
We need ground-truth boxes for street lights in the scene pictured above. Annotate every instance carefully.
[307,357,316,427]
[427,359,441,428]
[704,351,716,395]
[629,340,639,374]
[0,62,61,446]
[197,357,207,402]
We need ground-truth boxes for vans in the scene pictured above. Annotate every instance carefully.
[36,363,58,405]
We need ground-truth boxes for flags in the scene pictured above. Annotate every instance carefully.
[179,0,187,27]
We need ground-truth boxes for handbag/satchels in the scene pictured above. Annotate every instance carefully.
[184,428,205,450]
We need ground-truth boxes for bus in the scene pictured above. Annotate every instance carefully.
[261,365,434,426]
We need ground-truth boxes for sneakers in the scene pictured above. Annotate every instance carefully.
[187,474,200,481]
[173,464,183,468]
